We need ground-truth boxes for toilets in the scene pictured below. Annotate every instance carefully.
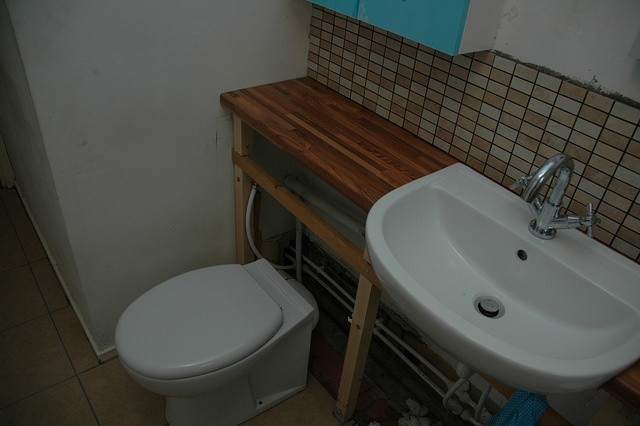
[115,257,319,425]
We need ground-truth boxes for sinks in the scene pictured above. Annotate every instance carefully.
[364,162,639,396]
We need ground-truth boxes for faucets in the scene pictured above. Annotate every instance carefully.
[520,154,575,231]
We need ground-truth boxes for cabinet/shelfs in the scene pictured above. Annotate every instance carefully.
[308,0,500,55]
[219,76,457,426]
